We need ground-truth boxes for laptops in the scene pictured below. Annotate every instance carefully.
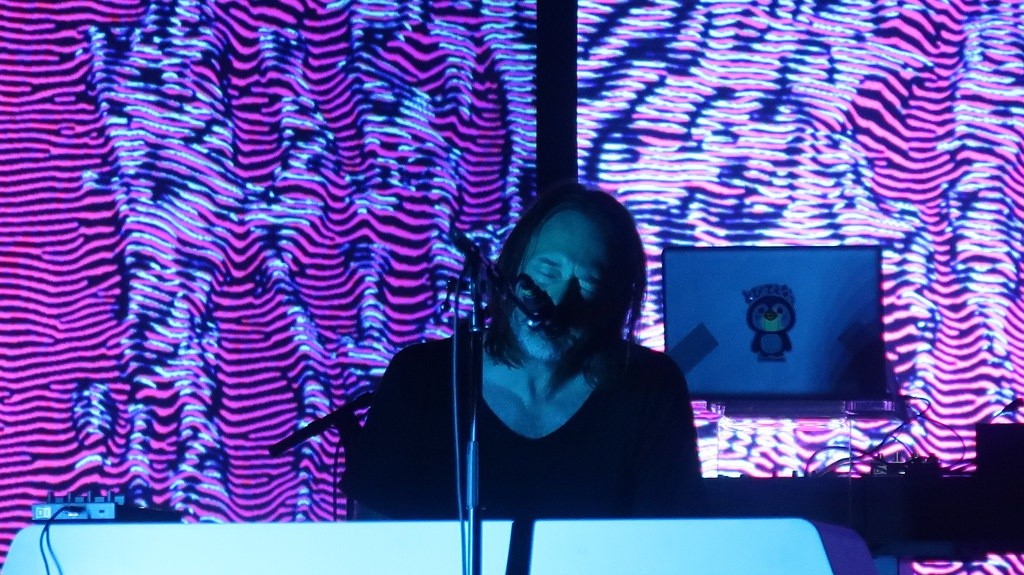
[662,244,892,399]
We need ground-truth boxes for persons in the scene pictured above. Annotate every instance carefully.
[335,184,703,521]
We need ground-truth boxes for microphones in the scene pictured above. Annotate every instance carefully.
[517,272,558,330]
